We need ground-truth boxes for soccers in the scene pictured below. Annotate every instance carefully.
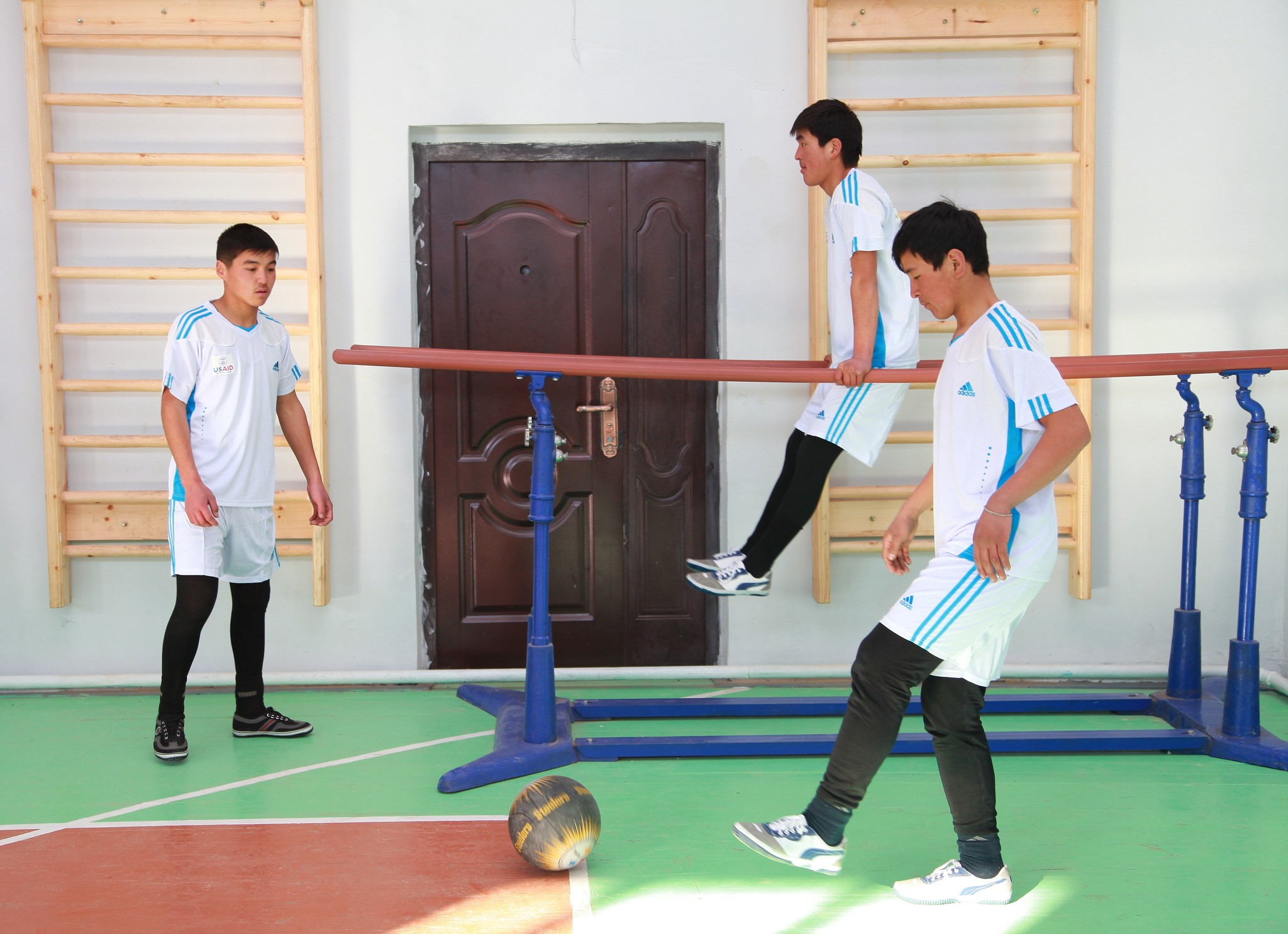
[508,776,602,872]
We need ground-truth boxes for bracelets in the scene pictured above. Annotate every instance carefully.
[983,505,1012,517]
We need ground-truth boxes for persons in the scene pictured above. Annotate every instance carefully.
[153,223,333,762]
[686,97,920,597]
[732,196,1093,904]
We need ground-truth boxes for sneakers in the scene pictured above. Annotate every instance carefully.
[687,548,747,572]
[731,816,846,875]
[893,858,1012,905]
[686,568,772,597]
[234,706,313,736]
[154,714,189,762]
